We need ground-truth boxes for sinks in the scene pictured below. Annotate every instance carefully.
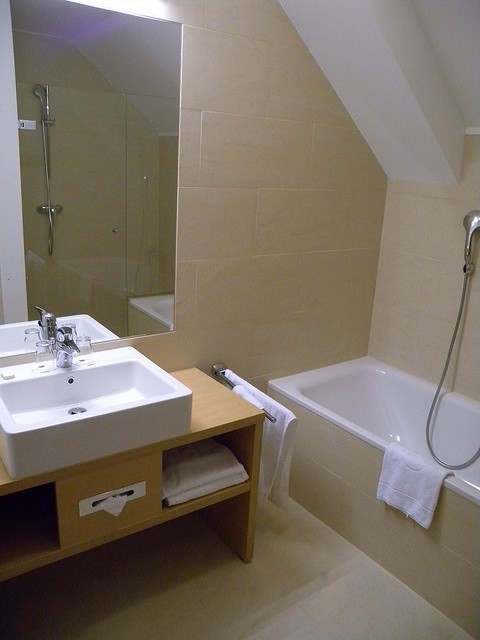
[0,358,179,426]
[0,313,120,358]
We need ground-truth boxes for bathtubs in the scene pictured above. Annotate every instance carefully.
[127,293,175,335]
[266,355,480,639]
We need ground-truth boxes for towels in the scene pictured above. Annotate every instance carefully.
[166,464,250,507]
[375,442,455,530]
[232,382,266,414]
[223,368,298,511]
[160,436,245,498]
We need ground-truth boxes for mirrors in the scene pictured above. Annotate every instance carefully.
[1,0,184,357]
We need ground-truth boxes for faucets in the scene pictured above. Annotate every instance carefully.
[56,326,80,368]
[34,304,57,349]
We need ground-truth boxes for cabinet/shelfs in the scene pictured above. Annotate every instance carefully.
[1,367,267,584]
